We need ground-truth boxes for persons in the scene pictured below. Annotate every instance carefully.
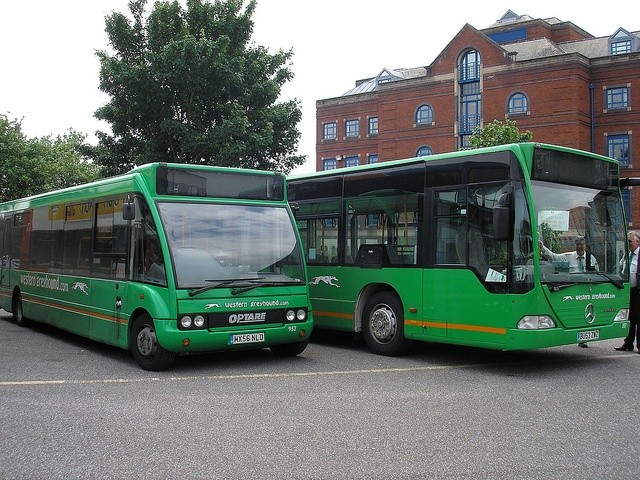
[612,231,640,354]
[533,234,600,349]
[48,245,57,254]
[138,236,160,282]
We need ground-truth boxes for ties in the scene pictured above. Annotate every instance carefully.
[629,253,634,268]
[578,256,584,271]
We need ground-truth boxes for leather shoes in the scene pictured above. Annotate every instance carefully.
[614,343,633,350]
[578,343,587,348]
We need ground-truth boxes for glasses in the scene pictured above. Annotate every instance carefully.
[628,240,633,242]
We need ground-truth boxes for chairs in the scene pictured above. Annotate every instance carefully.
[332,255,353,264]
[385,244,400,264]
[455,231,489,280]
[307,255,329,263]
[355,244,390,266]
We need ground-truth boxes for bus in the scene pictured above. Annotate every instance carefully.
[286,142,640,355]
[0,162,314,371]
